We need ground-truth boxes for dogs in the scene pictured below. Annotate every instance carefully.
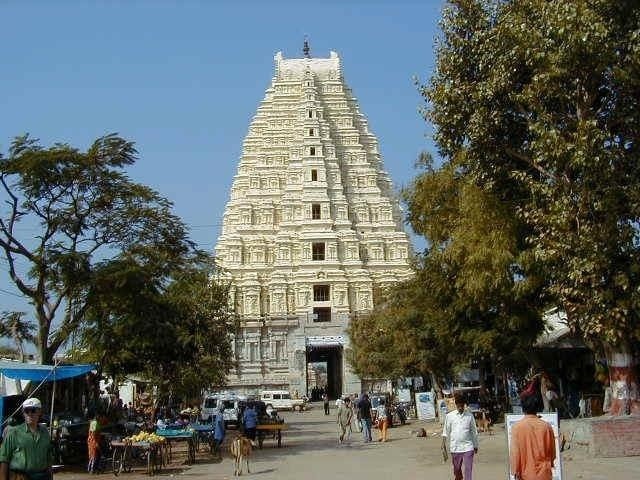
[476,417,491,433]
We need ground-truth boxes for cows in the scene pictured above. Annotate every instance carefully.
[231,435,257,476]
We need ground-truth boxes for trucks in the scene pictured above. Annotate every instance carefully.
[258,391,307,412]
[356,392,393,427]
[200,393,242,429]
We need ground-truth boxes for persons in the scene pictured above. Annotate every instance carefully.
[0,396,55,479]
[86,409,103,474]
[508,388,557,480]
[242,403,257,441]
[1,417,18,441]
[439,393,479,480]
[293,389,298,400]
[311,384,389,445]
[212,403,227,456]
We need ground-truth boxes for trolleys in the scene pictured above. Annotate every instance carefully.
[108,422,216,477]
[242,415,289,451]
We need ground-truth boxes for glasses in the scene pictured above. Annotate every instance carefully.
[24,408,39,414]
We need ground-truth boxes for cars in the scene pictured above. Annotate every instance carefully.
[334,393,359,409]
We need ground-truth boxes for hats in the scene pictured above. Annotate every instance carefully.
[23,397,42,410]
